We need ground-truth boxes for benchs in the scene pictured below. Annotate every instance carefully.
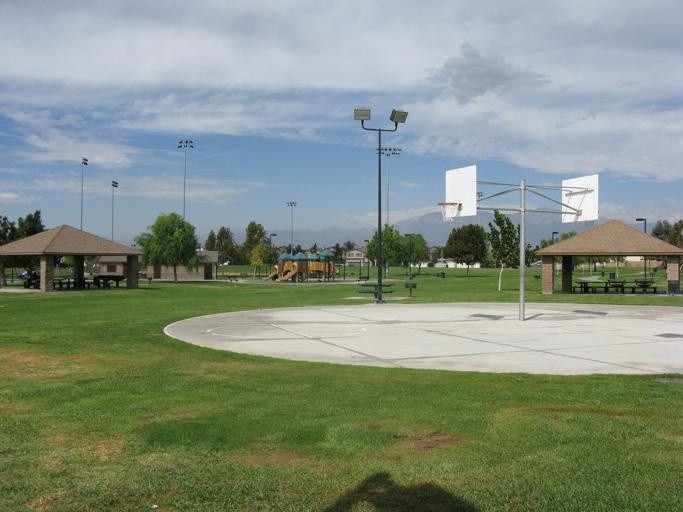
[24,281,110,289]
[570,286,659,293]
[359,289,396,298]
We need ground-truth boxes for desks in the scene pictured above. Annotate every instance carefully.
[575,282,655,294]
[52,278,89,289]
[362,284,393,298]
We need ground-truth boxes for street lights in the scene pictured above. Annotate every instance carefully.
[552,231,558,246]
[286,201,297,256]
[353,108,407,302]
[80,157,88,230]
[269,233,277,274]
[110,179,118,241]
[636,217,646,277]
[405,233,411,279]
[376,147,401,277]
[364,239,369,278]
[178,138,193,220]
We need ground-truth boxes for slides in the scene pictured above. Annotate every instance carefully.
[279,270,295,281]
[264,270,276,280]
